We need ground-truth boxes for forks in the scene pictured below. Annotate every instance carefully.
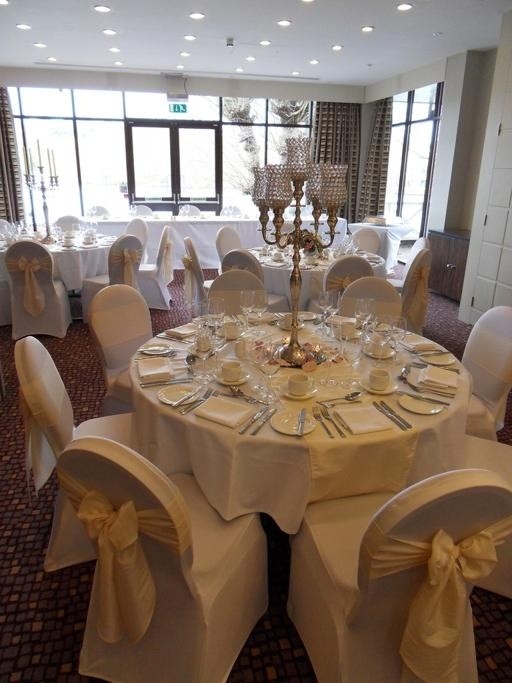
[178,387,214,412]
[312,406,335,439]
[181,390,220,415]
[320,406,347,439]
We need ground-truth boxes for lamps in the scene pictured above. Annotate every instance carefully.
[252,137,349,365]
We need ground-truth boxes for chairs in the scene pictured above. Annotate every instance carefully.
[87,283,153,417]
[460,305,512,442]
[15,336,193,573]
[56,435,269,682]
[287,467,512,683]
[463,433,512,599]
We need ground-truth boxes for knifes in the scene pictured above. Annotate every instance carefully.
[248,410,277,435]
[405,381,454,399]
[238,407,268,433]
[171,386,202,408]
[380,400,412,429]
[398,389,450,406]
[296,407,307,437]
[372,401,408,432]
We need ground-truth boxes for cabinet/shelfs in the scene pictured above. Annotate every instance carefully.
[427,229,470,302]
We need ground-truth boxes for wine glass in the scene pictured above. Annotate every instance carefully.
[5,221,98,242]
[194,290,408,409]
[140,312,454,370]
[263,231,360,255]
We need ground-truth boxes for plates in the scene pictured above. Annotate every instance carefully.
[156,384,204,405]
[0,231,118,251]
[246,245,380,269]
[270,410,317,434]
[398,393,444,415]
